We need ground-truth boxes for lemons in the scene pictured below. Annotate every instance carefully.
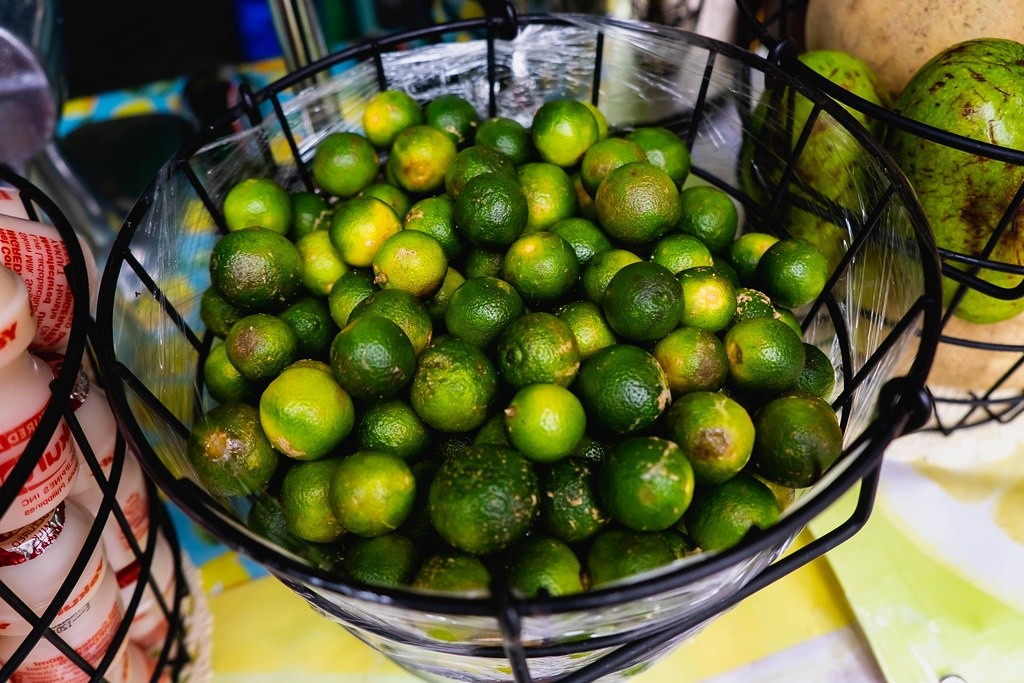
[179,93,842,600]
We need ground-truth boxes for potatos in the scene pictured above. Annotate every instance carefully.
[804,1,1023,392]
